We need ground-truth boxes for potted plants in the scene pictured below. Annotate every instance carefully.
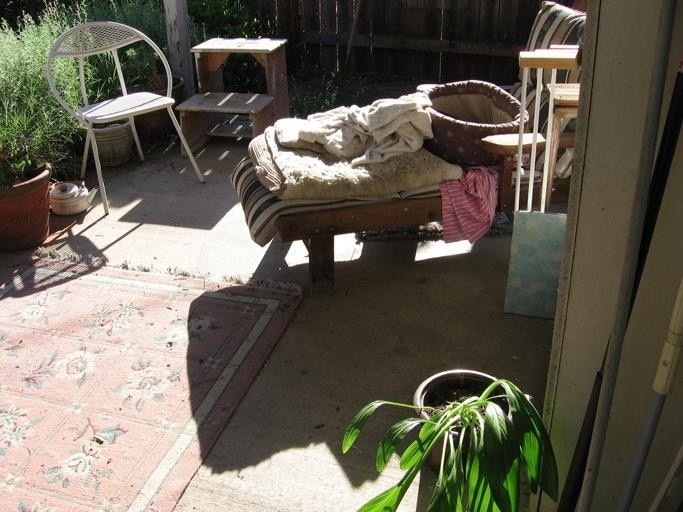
[120,48,185,143]
[0,134,53,252]
[341,369,557,512]
[92,76,134,167]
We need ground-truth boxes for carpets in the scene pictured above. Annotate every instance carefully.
[0,246,304,512]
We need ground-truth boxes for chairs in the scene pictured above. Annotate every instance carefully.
[46,20,205,215]
[230,1,586,298]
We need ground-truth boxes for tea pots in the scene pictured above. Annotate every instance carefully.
[49,182,99,216]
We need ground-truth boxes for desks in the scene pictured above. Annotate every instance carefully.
[547,84,583,214]
